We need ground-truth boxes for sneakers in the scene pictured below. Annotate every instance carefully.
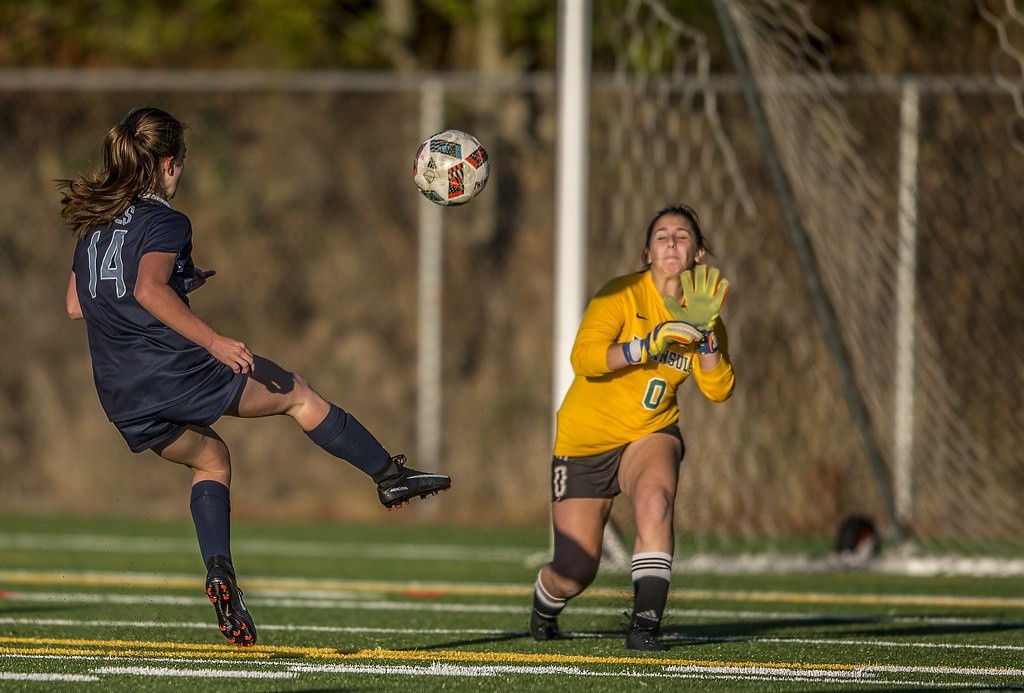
[529,590,561,641]
[618,611,666,651]
[376,454,451,511]
[203,554,257,647]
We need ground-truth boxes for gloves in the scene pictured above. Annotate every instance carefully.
[621,321,704,365]
[665,263,730,355]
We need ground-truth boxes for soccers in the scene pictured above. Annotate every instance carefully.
[414,127,493,206]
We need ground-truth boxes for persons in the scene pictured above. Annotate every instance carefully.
[523,203,738,653]
[64,105,452,648]
